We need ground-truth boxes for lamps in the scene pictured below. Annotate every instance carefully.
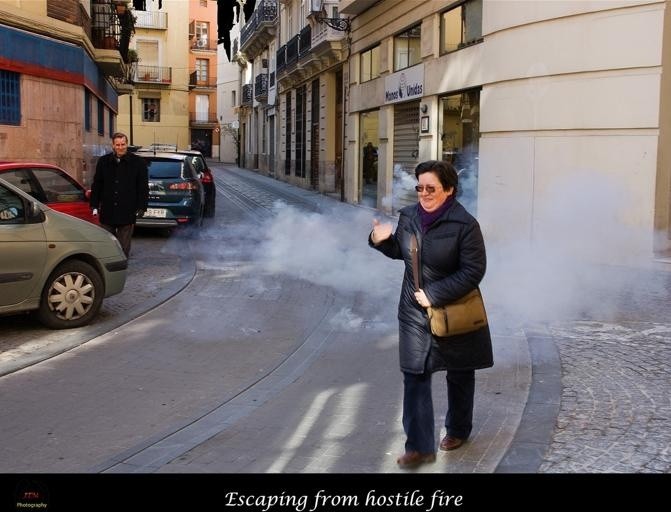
[309,0,352,32]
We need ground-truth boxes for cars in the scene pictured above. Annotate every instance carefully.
[0,160,106,230]
[0,176,128,331]
[156,150,219,219]
[121,151,206,238]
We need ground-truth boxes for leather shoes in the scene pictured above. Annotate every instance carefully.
[439,435,463,451]
[396,452,435,465]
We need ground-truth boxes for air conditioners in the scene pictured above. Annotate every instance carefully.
[197,39,205,48]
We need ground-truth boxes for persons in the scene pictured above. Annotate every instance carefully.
[367,160,494,468]
[90,132,150,259]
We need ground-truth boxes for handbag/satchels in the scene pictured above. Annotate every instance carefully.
[426,287,488,337]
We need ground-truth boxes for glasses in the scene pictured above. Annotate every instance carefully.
[414,185,435,193]
